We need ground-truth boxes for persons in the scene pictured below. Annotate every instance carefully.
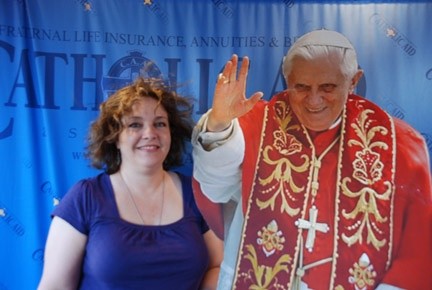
[34,76,225,290]
[191,29,432,290]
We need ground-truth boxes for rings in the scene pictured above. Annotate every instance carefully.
[218,73,229,84]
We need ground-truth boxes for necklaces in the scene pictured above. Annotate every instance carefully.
[119,170,166,225]
